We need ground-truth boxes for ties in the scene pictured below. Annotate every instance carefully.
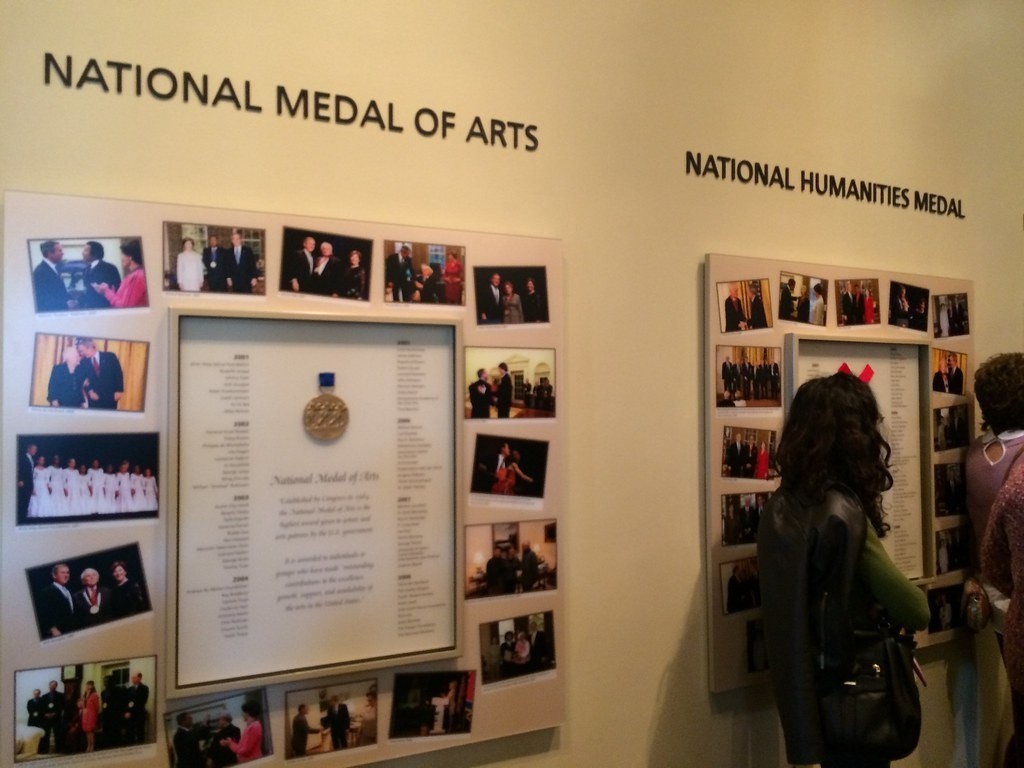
[92,358,99,374]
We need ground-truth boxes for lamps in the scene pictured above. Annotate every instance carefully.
[473,551,485,574]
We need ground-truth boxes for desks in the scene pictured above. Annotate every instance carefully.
[470,574,485,595]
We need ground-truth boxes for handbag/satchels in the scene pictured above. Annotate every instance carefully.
[823,629,921,760]
[960,577,990,629]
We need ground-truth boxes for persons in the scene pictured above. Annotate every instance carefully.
[34,240,148,309]
[481,274,541,324]
[486,541,539,595]
[468,363,553,418]
[781,279,825,324]
[177,233,259,294]
[386,245,463,304]
[478,441,533,494]
[756,371,931,768]
[842,280,874,324]
[292,691,376,756]
[290,238,365,301]
[891,285,928,329]
[44,564,143,637]
[724,432,770,613]
[487,622,549,681]
[964,353,1024,768]
[27,672,149,755]
[725,283,767,330]
[442,683,455,732]
[20,445,158,517]
[173,702,262,768]
[722,355,780,400]
[933,300,966,631]
[47,338,124,409]
